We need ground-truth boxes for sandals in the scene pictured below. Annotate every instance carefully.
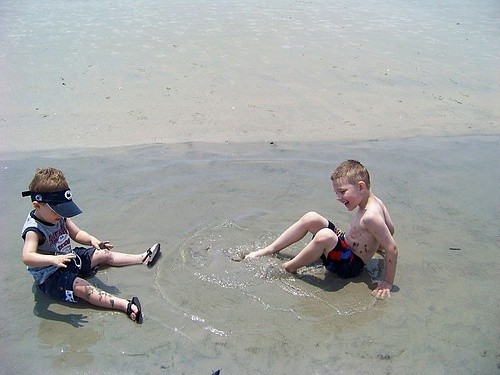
[142,243,160,267]
[127,296,143,325]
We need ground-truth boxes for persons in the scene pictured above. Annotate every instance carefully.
[21,168,161,324]
[241,159,399,299]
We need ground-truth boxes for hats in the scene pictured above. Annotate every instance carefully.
[22,188,83,218]
